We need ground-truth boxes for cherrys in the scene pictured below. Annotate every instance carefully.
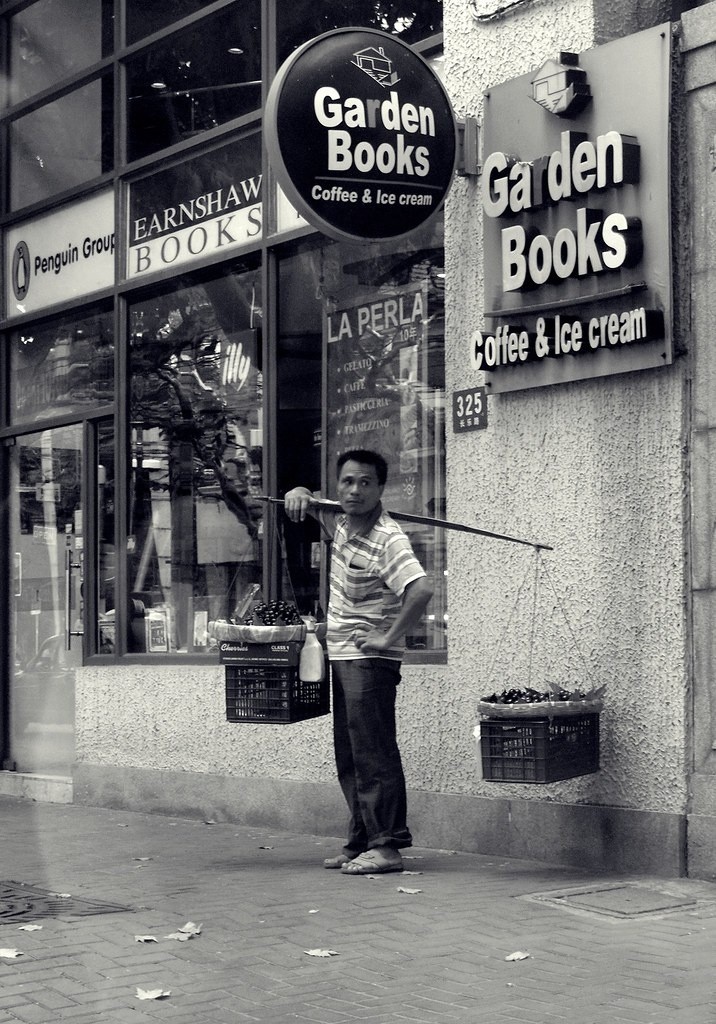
[231,596,300,626]
[486,685,582,709]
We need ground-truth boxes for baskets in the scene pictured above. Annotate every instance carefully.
[225,660,332,724]
[479,714,599,784]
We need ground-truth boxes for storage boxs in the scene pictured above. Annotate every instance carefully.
[478,710,601,784]
[217,641,331,726]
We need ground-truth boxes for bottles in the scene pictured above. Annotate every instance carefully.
[299,615,325,682]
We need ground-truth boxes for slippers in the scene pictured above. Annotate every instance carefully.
[323,851,404,875]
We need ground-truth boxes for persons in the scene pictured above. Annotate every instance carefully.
[288,450,433,875]
